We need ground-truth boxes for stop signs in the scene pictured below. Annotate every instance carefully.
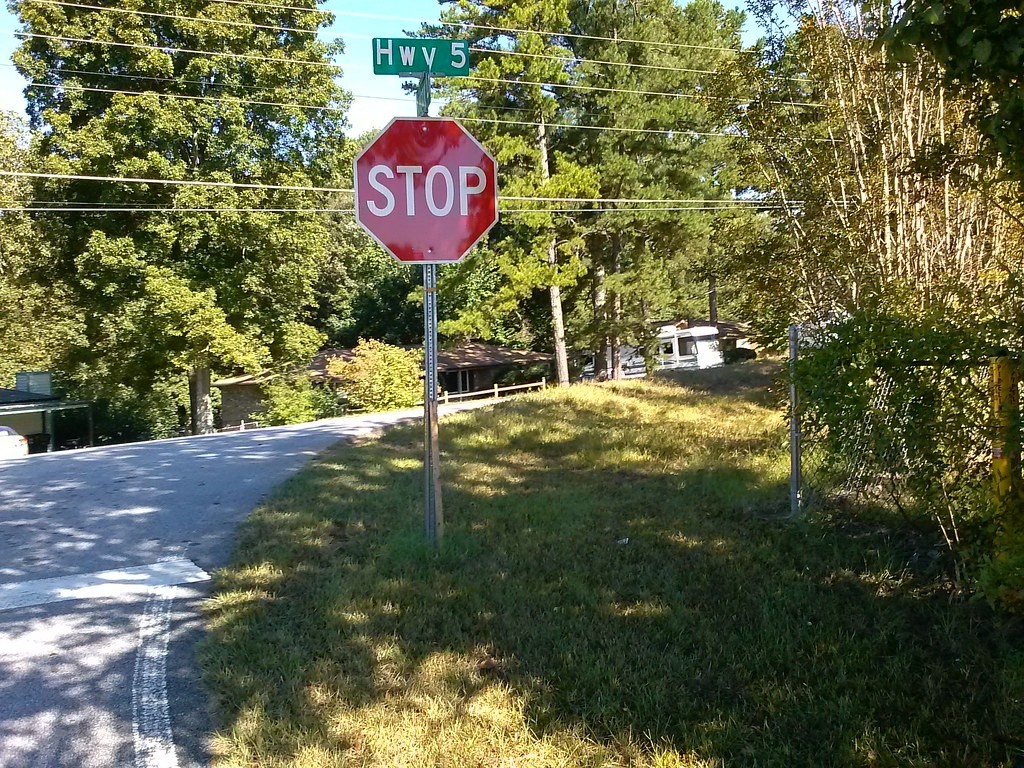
[353,115,500,266]
